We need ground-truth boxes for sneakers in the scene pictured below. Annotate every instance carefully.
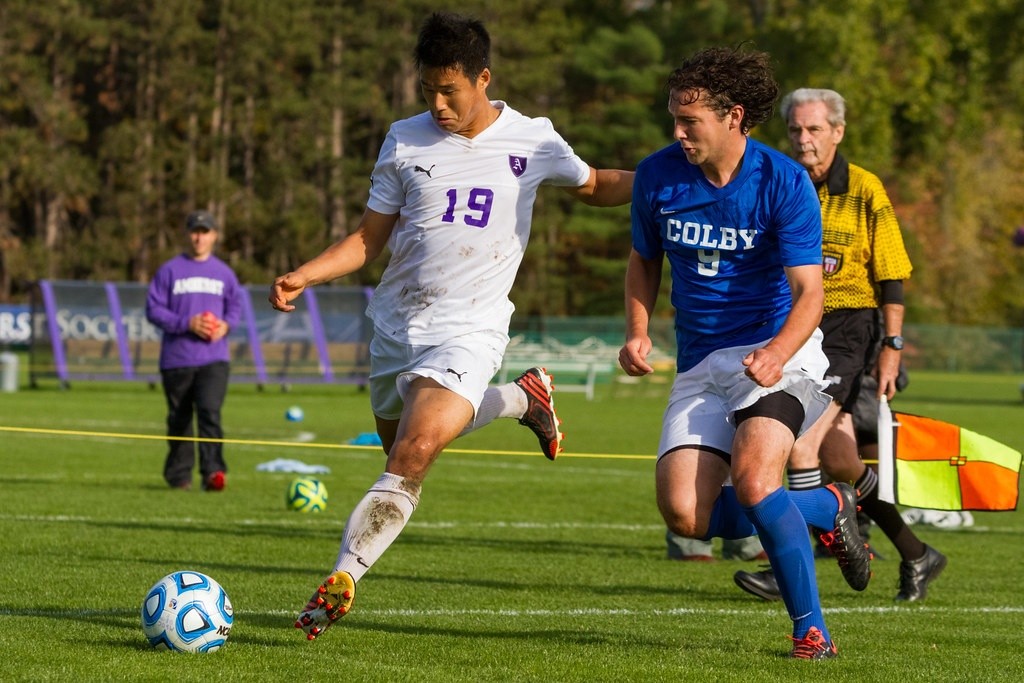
[792,628,838,662]
[824,480,873,591]
[295,570,354,639]
[171,473,227,491]
[514,367,564,461]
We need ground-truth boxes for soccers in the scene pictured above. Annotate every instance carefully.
[285,476,328,514]
[285,407,303,422]
[142,570,233,654]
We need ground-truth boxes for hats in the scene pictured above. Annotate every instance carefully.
[185,210,217,230]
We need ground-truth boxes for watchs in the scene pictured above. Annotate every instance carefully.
[879,334,905,351]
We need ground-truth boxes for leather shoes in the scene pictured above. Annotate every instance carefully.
[893,544,946,601]
[734,568,785,602]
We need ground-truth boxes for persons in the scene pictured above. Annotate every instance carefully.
[266,8,656,641]
[733,82,953,609]
[616,49,874,659]
[141,211,247,490]
